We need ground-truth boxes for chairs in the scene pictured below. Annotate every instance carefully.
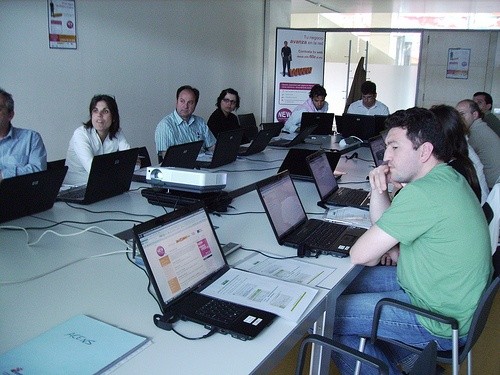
[296,333,438,374]
[47,158,66,169]
[259,122,284,143]
[238,113,258,129]
[359,275,500,375]
[131,146,152,173]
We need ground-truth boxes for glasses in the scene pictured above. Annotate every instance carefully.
[222,98,236,105]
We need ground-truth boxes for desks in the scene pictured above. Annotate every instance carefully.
[0,129,402,374]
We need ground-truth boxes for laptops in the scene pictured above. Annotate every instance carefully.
[0,166,68,224]
[336,113,389,167]
[306,149,372,211]
[132,139,205,183]
[132,201,278,340]
[253,169,369,259]
[302,112,335,135]
[194,128,244,169]
[56,147,139,206]
[237,129,276,156]
[268,124,318,147]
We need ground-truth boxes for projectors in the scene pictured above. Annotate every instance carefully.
[146,166,227,194]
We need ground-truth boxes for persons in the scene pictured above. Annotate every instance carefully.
[281,40,292,77]
[347,81,389,116]
[281,84,329,134]
[454,99,500,191]
[154,85,216,164]
[57,94,141,196]
[472,92,500,140]
[0,88,48,180]
[304,106,494,375]
[206,88,251,147]
[426,103,490,215]
